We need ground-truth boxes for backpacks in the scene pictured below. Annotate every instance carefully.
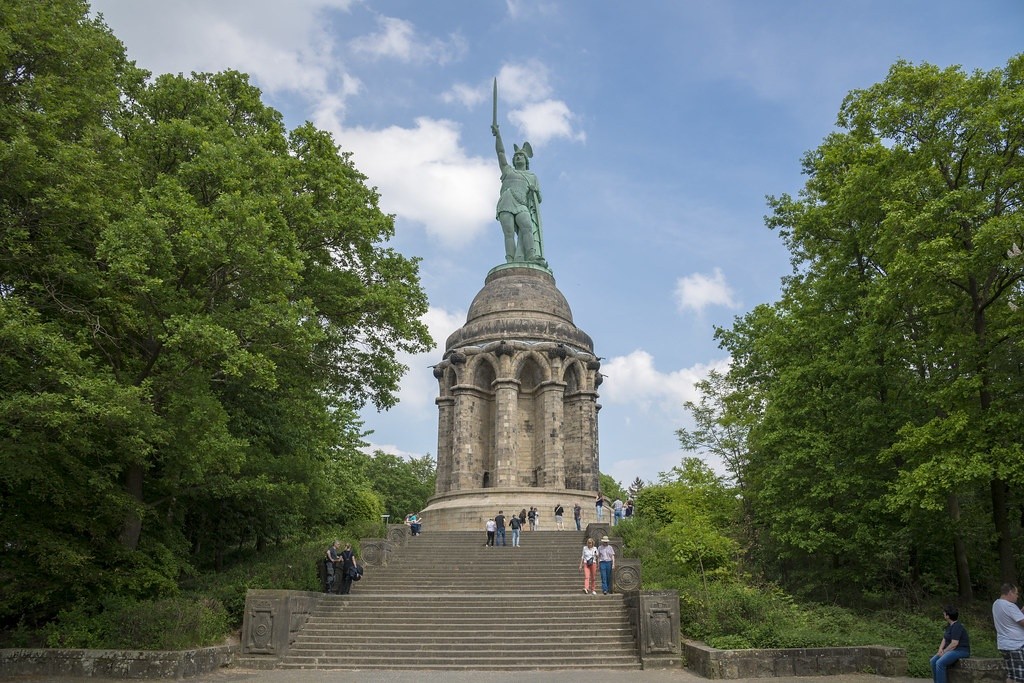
[520,512,525,519]
[512,519,518,529]
[530,511,534,518]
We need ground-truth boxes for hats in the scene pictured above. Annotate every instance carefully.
[601,535,610,542]
[522,509,526,512]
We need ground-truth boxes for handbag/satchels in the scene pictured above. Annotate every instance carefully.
[587,557,594,566]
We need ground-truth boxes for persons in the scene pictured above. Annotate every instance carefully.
[597,536,615,594]
[930,606,971,683]
[519,506,539,531]
[554,504,564,531]
[509,515,521,548]
[323,541,359,594]
[991,583,1024,683]
[574,502,582,531]
[595,492,604,523]
[579,538,599,595]
[490,123,548,269]
[485,510,506,548]
[404,511,423,537]
[611,496,634,526]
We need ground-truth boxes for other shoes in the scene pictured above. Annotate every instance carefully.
[604,591,607,595]
[592,590,597,595]
[516,545,520,548]
[583,588,588,594]
